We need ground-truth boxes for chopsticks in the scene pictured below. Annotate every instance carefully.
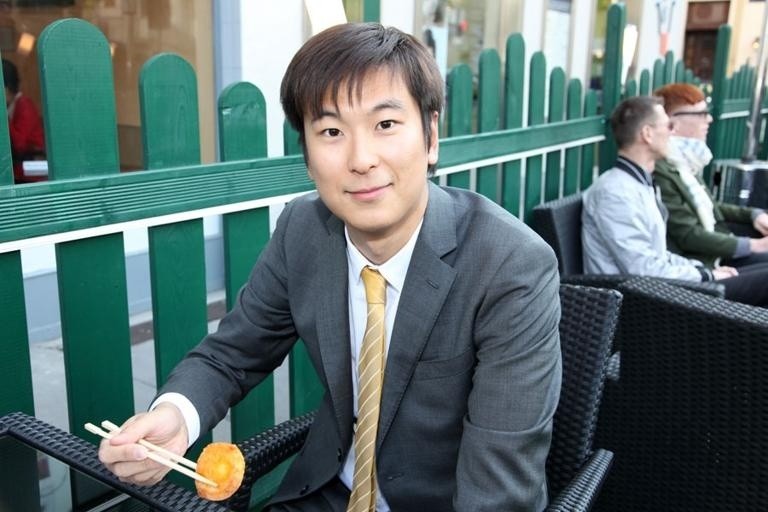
[84,418,218,488]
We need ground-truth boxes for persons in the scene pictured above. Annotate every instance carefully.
[96,19,563,511]
[579,93,767,310]
[424,0,457,88]
[646,84,767,268]
[1,60,45,183]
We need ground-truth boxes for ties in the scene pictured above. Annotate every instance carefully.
[346,268,387,512]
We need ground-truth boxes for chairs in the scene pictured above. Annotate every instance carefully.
[220,286,624,510]
[533,192,726,381]
[616,276,767,512]
